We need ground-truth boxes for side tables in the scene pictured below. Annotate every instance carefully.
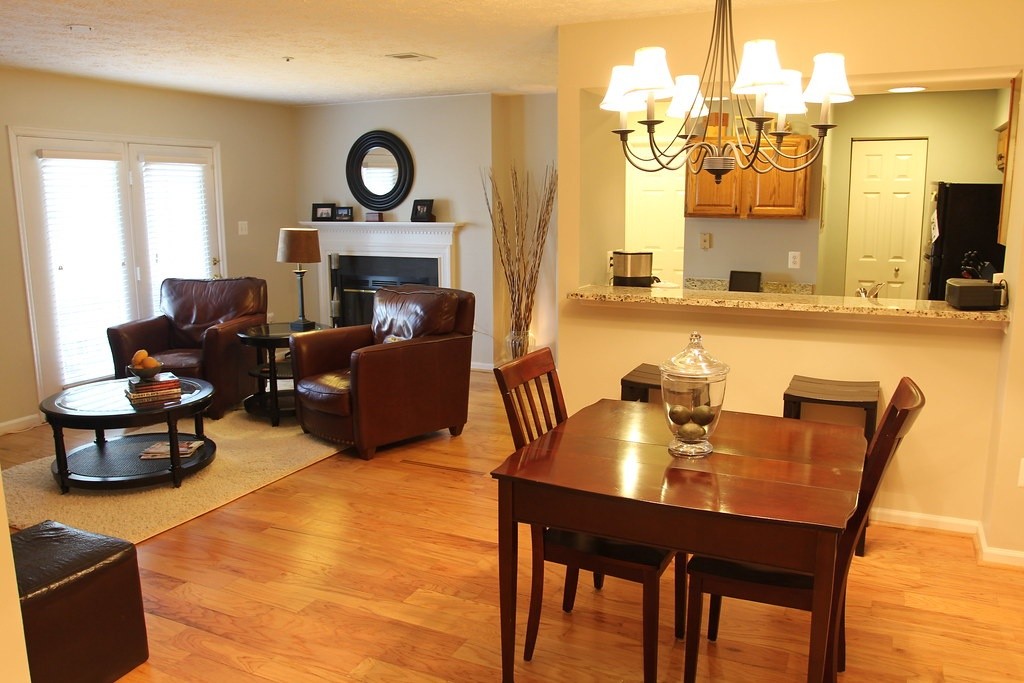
[234,323,332,427]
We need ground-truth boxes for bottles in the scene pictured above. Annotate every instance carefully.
[659,332,730,457]
[660,448,720,513]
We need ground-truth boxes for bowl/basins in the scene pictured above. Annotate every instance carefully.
[650,282,680,289]
[127,362,165,381]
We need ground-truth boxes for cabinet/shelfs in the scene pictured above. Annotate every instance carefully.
[684,135,813,221]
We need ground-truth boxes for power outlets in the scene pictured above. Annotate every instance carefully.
[788,251,800,269]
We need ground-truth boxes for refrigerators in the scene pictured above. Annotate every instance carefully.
[927,181,1006,301]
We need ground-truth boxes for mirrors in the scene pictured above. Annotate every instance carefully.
[346,129,414,211]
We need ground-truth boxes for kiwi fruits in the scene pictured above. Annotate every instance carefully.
[668,404,716,440]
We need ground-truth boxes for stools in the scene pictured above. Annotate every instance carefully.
[620,362,708,408]
[781,375,880,556]
[9,520,150,683]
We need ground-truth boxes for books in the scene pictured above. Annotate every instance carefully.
[125,372,181,405]
[142,440,204,459]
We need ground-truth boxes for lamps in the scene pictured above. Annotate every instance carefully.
[597,0,855,184]
[277,227,322,332]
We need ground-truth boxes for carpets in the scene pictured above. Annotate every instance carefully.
[0,409,353,545]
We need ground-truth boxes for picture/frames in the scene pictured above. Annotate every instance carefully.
[336,206,353,221]
[412,199,437,223]
[311,203,336,221]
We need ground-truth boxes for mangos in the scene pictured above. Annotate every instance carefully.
[131,350,160,369]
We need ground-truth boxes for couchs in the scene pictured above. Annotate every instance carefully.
[106,277,269,420]
[289,284,475,460]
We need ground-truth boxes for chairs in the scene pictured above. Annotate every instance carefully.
[494,348,689,683]
[683,375,927,683]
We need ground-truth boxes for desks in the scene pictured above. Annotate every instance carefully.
[39,376,216,493]
[490,398,869,683]
